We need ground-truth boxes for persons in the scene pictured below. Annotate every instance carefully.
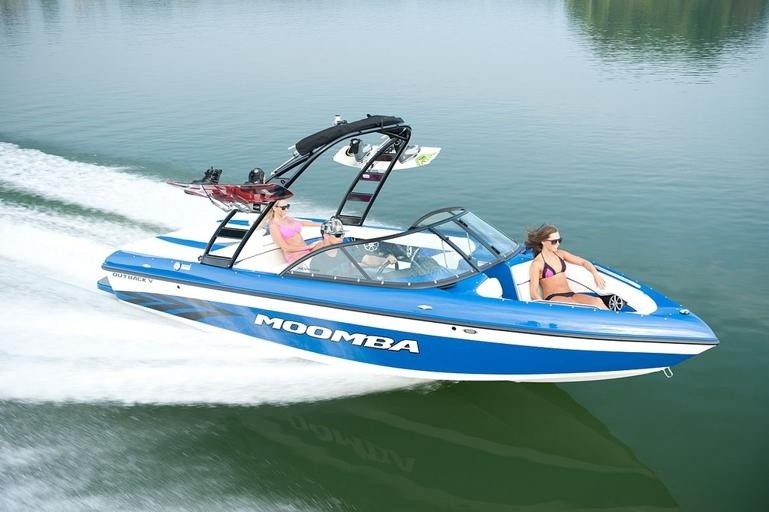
[524,223,610,310]
[310,217,398,278]
[258,199,321,266]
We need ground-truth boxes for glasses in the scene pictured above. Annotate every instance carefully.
[332,235,341,237]
[281,204,290,210]
[546,239,561,244]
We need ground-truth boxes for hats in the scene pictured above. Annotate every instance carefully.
[321,218,351,235]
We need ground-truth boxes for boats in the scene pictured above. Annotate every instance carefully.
[92,113,720,383]
[207,385,680,512]
